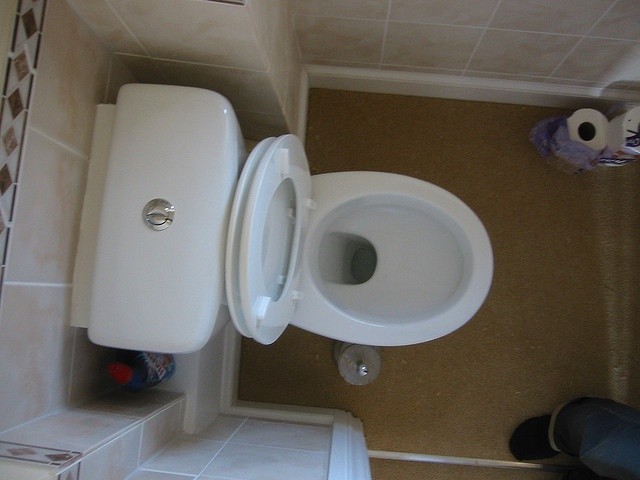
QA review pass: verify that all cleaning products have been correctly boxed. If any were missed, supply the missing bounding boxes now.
[106,354,175,392]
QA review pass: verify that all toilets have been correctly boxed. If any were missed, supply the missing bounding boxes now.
[87,83,494,356]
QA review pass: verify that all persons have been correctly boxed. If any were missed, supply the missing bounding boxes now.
[509,393,640,479]
